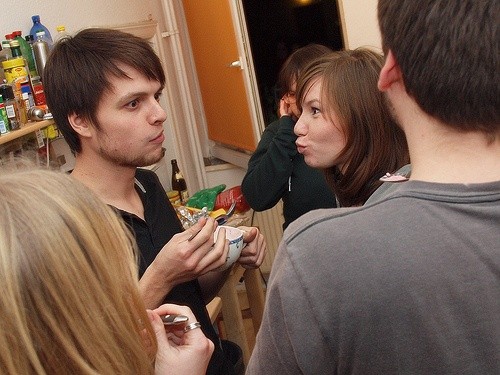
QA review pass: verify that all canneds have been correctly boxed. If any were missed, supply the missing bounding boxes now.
[0,58,36,137]
[166,191,181,220]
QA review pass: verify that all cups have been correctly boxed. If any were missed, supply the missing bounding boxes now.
[202,226,248,274]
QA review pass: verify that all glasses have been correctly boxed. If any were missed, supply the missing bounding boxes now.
[281,92,296,103]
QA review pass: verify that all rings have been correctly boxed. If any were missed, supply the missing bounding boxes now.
[183,322,201,332]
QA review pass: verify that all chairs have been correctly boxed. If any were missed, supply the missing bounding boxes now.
[200,217,267,368]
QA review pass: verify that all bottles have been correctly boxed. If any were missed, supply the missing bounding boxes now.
[33,38,51,78]
[55,26,71,47]
[35,31,55,54]
[0,31,44,133]
[170,159,189,205]
[28,15,53,57]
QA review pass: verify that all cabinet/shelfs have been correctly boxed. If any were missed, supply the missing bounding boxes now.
[0,118,55,169]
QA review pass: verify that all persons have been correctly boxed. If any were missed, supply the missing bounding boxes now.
[246,0,500,375]
[0,141,216,375]
[43,28,270,375]
[240,43,340,229]
[293,45,412,209]
